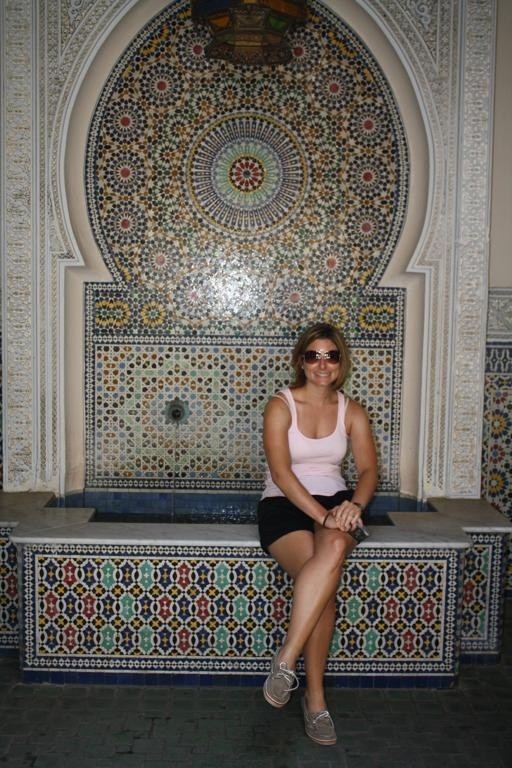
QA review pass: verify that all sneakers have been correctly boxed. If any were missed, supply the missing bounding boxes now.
[303,696,338,746]
[263,647,300,709]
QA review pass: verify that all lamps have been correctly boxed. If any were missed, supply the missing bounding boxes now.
[191,0,310,65]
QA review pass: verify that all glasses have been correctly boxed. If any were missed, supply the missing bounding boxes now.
[301,350,340,364]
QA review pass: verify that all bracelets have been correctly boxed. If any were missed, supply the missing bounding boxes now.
[322,512,331,528]
[354,502,363,510]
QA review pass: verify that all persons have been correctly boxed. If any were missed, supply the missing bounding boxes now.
[256,324,379,746]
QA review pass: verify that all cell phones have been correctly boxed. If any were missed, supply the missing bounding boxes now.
[348,525,369,544]
